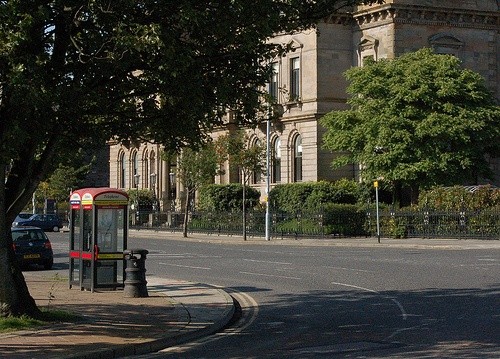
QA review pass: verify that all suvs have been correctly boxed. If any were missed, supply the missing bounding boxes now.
[16,214,63,232]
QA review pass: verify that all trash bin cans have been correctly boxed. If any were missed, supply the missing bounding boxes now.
[122,249,149,297]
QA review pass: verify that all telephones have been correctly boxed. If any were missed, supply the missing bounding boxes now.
[87,230,102,250]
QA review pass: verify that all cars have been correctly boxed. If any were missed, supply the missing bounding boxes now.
[12,213,35,227]
[10,227,54,269]
